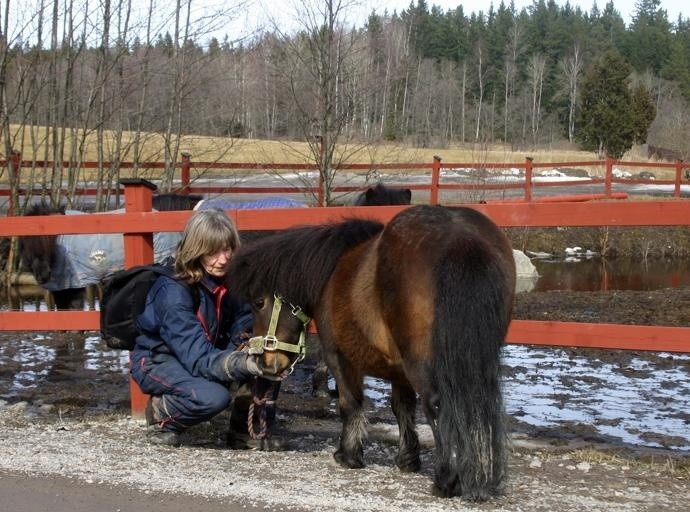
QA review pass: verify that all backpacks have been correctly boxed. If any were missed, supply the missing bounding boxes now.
[99,256,201,351]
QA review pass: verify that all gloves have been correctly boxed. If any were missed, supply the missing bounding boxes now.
[246,354,289,382]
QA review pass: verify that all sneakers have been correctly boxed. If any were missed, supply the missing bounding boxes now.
[145,394,181,448]
[227,427,290,451]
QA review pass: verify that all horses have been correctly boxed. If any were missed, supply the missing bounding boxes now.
[223,202,518,501]
[16,204,183,310]
[330,183,414,207]
[149,191,310,243]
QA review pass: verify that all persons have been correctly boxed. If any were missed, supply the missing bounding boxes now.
[130,207,289,452]
